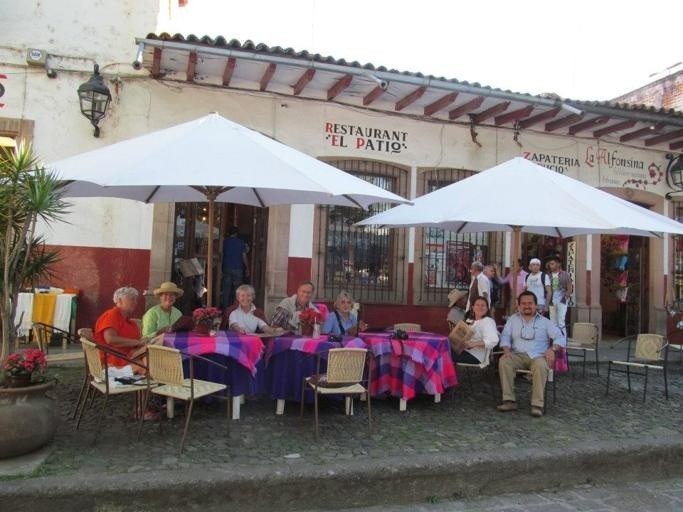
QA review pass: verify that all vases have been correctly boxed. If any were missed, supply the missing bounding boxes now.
[9,373,30,386]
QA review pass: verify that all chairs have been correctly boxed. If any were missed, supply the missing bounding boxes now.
[393,323,670,422]
[72,318,236,452]
[299,347,375,442]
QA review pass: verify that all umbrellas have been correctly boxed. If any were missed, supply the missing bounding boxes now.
[350,156,682,318]
[2,108,415,307]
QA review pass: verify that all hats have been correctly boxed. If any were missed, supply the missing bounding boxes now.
[153,281,184,298]
[528,258,541,271]
[448,288,469,308]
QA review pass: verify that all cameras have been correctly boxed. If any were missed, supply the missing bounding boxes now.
[328,335,342,342]
[394,329,408,339]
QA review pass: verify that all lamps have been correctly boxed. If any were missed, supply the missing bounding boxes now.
[77,64,112,138]
[665,152,683,200]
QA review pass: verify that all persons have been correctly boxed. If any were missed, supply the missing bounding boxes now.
[221,226,250,312]
[269,281,324,332]
[446,243,573,417]
[141,281,185,410]
[91,287,162,422]
[322,291,367,336]
[219,284,282,337]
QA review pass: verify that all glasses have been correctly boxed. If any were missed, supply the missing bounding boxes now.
[520,327,536,340]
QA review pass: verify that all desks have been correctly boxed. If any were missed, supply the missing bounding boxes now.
[14,293,79,350]
[165,330,459,426]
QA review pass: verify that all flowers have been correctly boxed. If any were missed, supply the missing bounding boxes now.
[299,302,329,322]
[3,348,47,374]
[193,308,222,323]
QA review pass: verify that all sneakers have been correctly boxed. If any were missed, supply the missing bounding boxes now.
[497,401,518,411]
[531,406,543,417]
[135,407,159,420]
